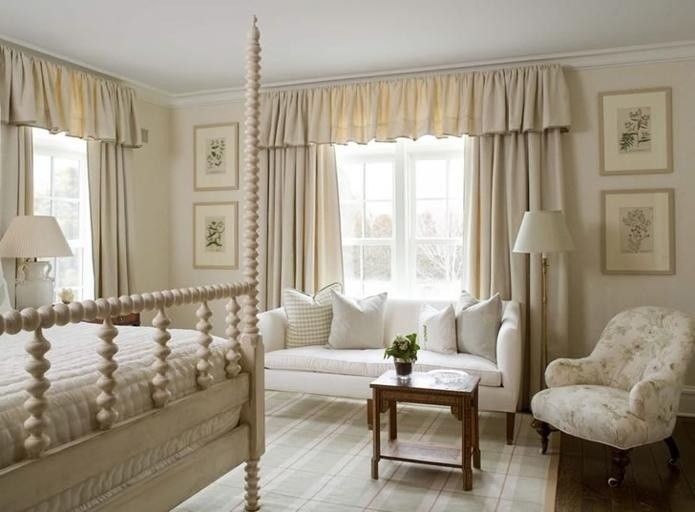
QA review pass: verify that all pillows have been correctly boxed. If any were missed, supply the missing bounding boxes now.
[279,281,390,352]
[418,287,504,365]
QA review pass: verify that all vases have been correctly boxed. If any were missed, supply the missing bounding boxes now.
[393,356,412,377]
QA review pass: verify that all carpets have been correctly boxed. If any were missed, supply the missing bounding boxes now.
[167,390,566,511]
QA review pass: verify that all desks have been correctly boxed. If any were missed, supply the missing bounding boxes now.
[368,369,483,491]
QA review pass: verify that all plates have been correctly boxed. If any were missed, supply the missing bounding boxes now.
[428,370,469,381]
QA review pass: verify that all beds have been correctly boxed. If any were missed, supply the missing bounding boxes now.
[1,14,269,512]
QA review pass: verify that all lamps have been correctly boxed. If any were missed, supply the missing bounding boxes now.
[512,208,577,390]
[2,214,73,313]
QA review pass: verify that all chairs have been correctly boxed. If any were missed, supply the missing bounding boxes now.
[529,301,695,491]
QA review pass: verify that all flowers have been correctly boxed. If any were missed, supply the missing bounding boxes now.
[383,331,420,364]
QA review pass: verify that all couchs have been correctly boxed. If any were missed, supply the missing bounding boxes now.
[253,290,527,448]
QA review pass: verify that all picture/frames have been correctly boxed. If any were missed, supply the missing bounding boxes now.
[596,85,676,178]
[191,120,241,194]
[191,201,241,270]
[598,187,678,276]
